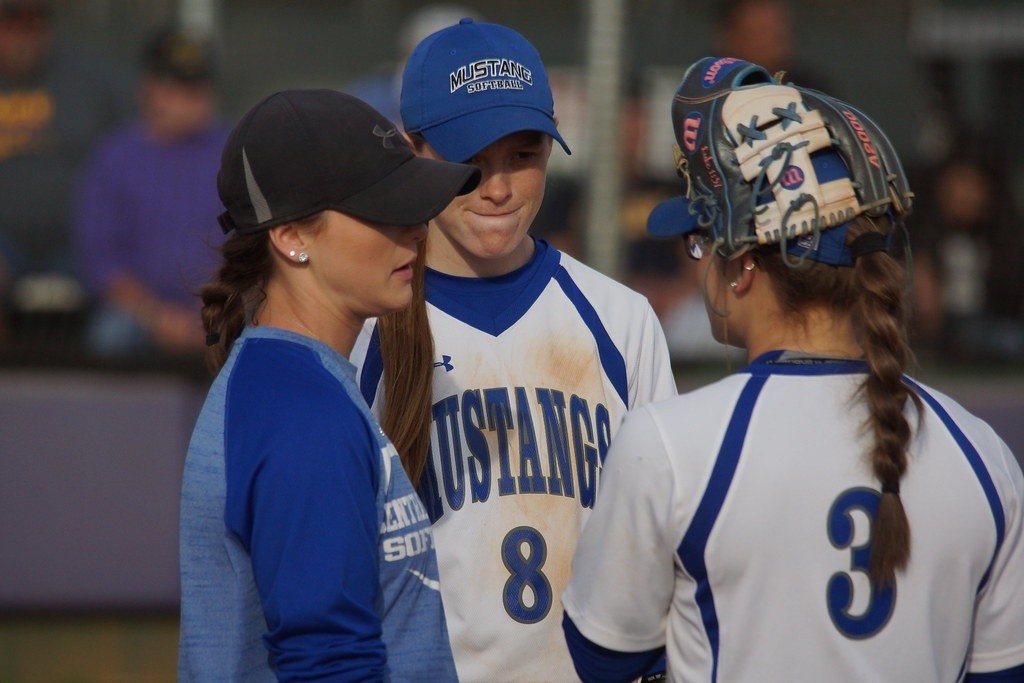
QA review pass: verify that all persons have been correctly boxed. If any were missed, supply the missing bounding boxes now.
[559,56,1024,683]
[721,1,944,350]
[79,39,245,373]
[175,88,483,683]
[350,18,677,683]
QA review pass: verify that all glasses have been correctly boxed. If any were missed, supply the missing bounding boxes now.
[683,230,712,260]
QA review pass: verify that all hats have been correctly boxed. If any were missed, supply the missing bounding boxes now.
[646,149,894,266]
[146,30,213,82]
[216,88,481,234]
[400,17,572,165]
